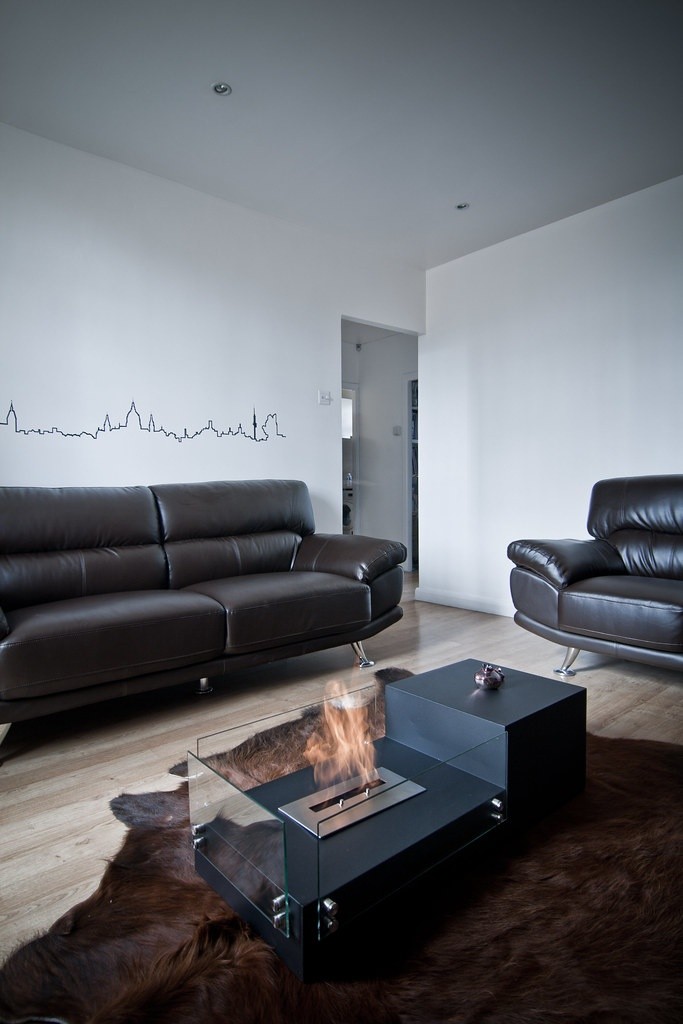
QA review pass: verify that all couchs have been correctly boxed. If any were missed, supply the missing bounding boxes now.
[504,472,683,680]
[0,477,411,744]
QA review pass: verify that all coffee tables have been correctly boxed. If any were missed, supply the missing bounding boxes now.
[184,657,598,986]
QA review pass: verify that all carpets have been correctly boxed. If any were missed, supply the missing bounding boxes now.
[0,667,683,1024]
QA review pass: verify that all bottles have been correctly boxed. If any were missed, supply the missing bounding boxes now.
[346,473,352,486]
[474,663,501,689]
